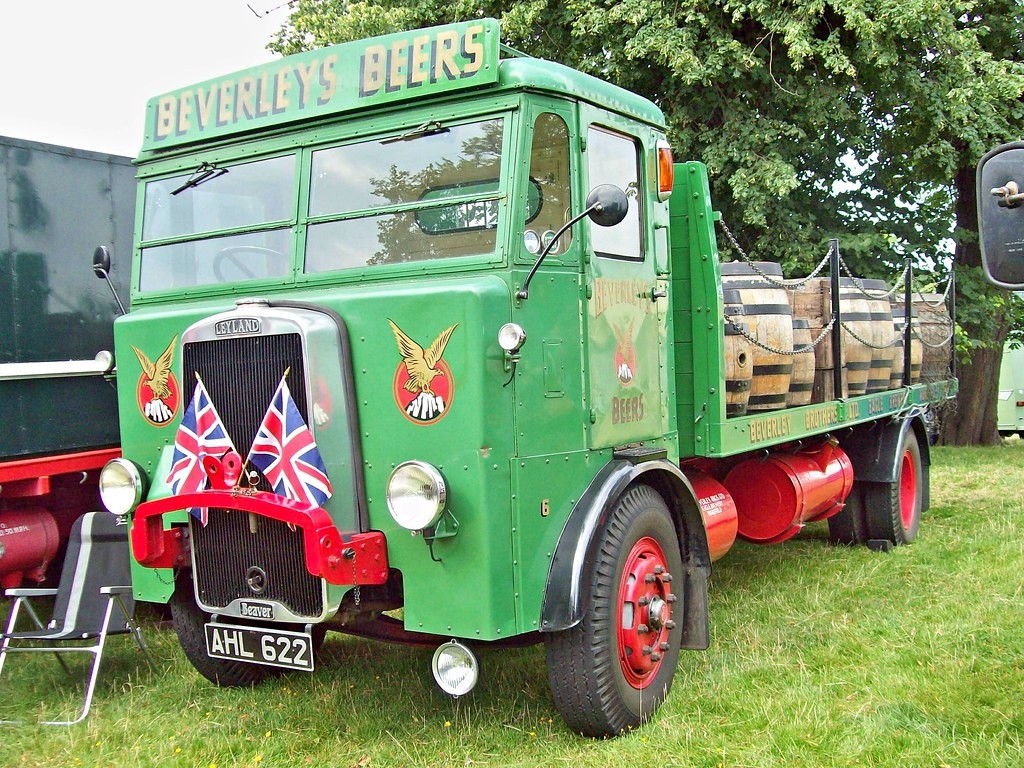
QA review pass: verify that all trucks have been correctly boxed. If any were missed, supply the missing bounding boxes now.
[93,18,959,740]
[0,137,135,597]
[960,322,1024,440]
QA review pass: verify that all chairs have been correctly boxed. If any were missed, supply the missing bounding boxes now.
[0,512,160,727]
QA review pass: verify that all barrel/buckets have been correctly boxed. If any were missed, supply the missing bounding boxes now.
[719,262,793,416]
[721,290,753,419]
[891,309,923,385]
[860,279,896,394]
[895,294,952,383]
[787,320,815,407]
[889,323,905,389]
[795,277,872,399]
